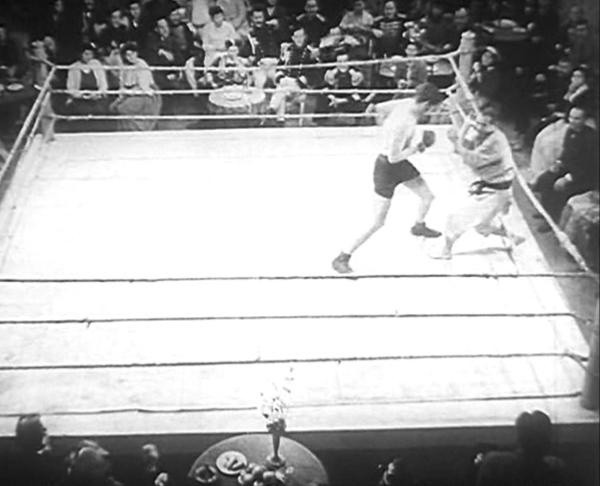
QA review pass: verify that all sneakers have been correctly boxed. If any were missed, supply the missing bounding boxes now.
[505,233,526,252]
[332,251,358,281]
[428,247,452,260]
[411,221,441,238]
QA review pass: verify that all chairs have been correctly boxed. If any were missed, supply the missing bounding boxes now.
[272,44,311,125]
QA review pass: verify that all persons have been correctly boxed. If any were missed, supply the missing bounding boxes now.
[427,107,528,261]
[331,82,444,281]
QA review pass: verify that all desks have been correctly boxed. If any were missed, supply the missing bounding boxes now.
[202,83,268,128]
[185,434,330,485]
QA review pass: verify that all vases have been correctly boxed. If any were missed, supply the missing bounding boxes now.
[265,422,290,466]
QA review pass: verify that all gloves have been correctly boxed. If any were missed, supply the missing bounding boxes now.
[418,130,435,153]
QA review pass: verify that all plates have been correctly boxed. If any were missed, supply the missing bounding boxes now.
[215,450,248,475]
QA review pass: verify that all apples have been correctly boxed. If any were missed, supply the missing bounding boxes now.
[240,463,285,486]
